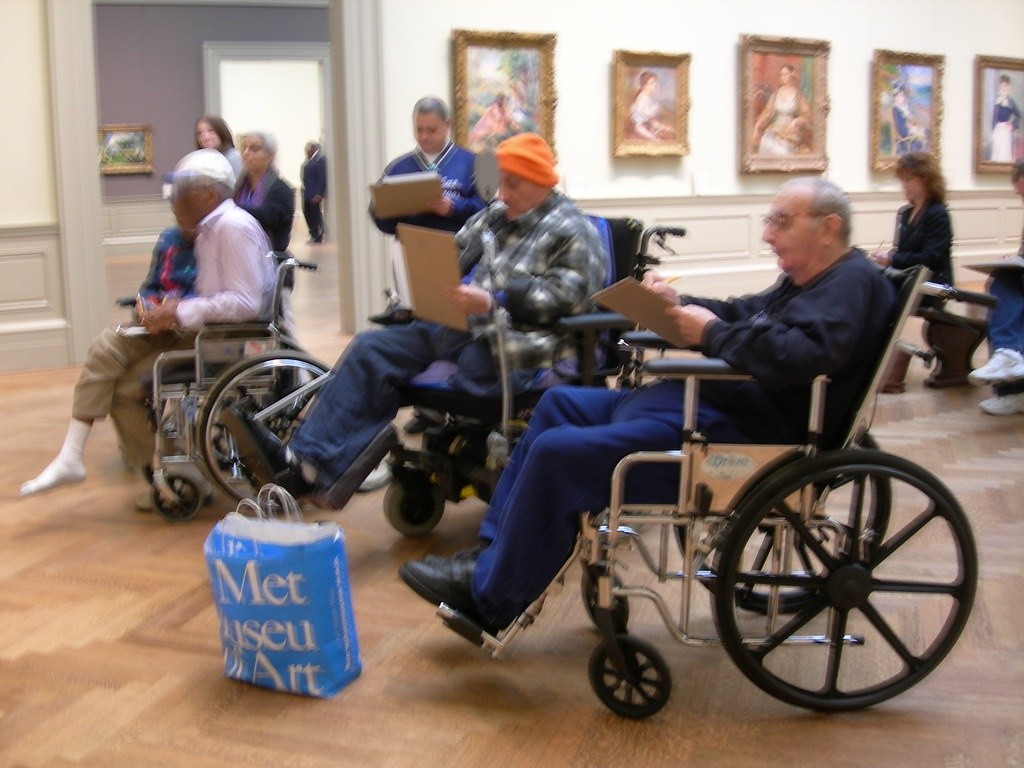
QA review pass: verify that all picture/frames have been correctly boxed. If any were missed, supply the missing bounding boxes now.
[613,47,693,157]
[739,31,831,173]
[971,53,1023,174]
[97,123,153,175]
[452,29,558,165]
[869,49,945,172]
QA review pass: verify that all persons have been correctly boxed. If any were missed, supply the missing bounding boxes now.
[368,96,487,234]
[17,146,277,511]
[299,141,326,246]
[223,134,606,512]
[230,128,295,253]
[877,151,955,310]
[195,115,243,176]
[968,160,1024,414]
[401,174,891,635]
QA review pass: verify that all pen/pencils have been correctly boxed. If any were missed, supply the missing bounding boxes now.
[161,295,166,306]
[647,276,680,291]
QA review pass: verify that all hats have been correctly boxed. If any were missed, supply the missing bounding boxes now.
[496,132,557,188]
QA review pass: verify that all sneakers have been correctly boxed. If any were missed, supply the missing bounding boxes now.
[968,347,1024,386]
[980,392,1024,415]
[424,545,483,569]
[400,559,499,637]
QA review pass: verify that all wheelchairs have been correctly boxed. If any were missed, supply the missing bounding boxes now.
[434,259,1003,722]
[212,214,692,549]
[114,249,338,524]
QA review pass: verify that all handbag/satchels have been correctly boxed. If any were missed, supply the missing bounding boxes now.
[205,483,362,700]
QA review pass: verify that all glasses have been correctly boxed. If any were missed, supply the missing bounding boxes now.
[764,211,848,232]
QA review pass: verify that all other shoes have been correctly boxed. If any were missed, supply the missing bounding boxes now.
[357,456,393,490]
[403,414,436,434]
[224,406,310,505]
